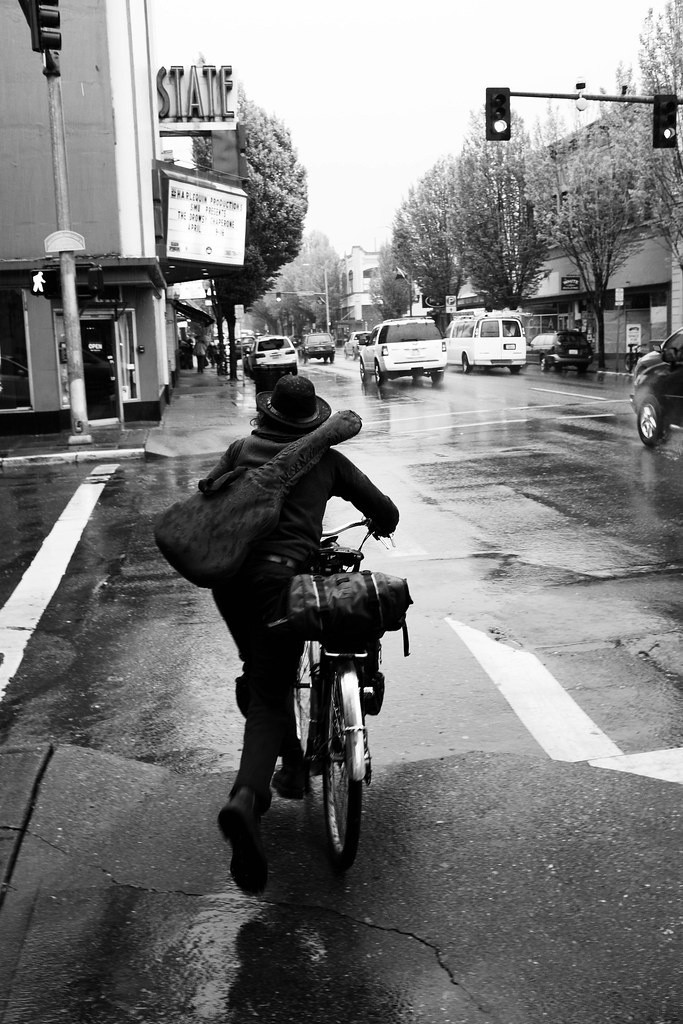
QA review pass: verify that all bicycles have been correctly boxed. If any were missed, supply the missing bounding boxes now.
[294,514,383,871]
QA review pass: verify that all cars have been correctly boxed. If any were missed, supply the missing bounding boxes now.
[344,331,373,361]
[1,354,31,405]
[290,334,303,356]
[302,333,335,363]
[630,347,683,447]
[246,336,298,379]
[58,347,114,402]
[235,333,262,372]
[632,326,683,380]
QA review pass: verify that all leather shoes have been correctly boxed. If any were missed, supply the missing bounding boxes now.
[271,739,315,803]
[216,786,275,897]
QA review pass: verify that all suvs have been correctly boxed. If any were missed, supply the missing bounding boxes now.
[526,330,593,375]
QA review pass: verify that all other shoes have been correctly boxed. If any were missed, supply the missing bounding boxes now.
[198,370,203,375]
[218,372,229,377]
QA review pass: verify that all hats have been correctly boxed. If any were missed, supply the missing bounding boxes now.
[255,375,333,439]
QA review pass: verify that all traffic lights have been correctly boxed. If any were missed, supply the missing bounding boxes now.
[29,269,59,296]
[485,87,512,142]
[652,93,679,148]
[276,291,282,303]
[33,0,64,53]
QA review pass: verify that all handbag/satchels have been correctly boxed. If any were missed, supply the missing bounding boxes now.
[288,570,414,652]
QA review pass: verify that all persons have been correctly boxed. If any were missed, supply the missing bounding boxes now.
[178,338,218,374]
[204,377,400,896]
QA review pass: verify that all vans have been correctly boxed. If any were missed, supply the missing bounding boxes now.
[359,317,447,385]
[444,316,527,374]
